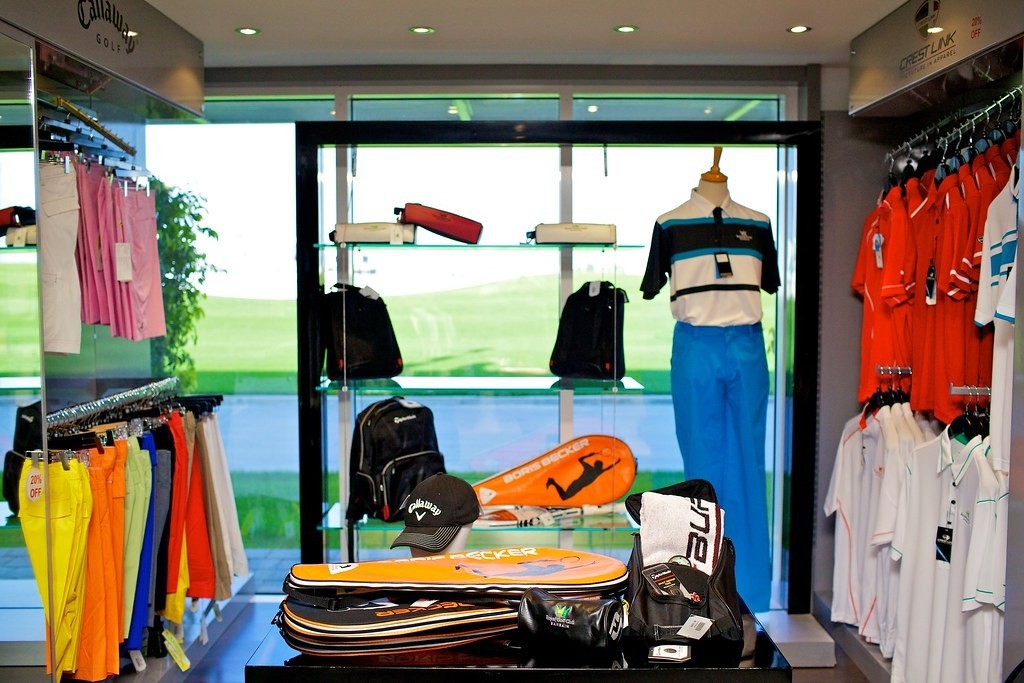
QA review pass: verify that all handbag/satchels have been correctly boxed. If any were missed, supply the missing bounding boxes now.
[626,479,745,667]
[329,221,417,245]
[394,202,483,244]
[526,223,616,244]
[517,586,624,652]
[549,281,630,380]
[322,283,403,380]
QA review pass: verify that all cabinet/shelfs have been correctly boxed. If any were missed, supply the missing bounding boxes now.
[292,121,836,675]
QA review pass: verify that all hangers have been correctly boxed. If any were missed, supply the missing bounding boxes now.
[947,386,993,447]
[866,362,916,417]
[37,100,155,197]
[21,379,224,471]
[880,91,1023,197]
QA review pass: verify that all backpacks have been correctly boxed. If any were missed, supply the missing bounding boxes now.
[344,395,447,522]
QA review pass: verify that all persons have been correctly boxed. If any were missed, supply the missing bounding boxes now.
[638,147,782,615]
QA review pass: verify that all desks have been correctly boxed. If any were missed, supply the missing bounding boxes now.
[246,597,792,681]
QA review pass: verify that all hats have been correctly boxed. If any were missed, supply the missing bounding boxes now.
[390,473,479,553]
